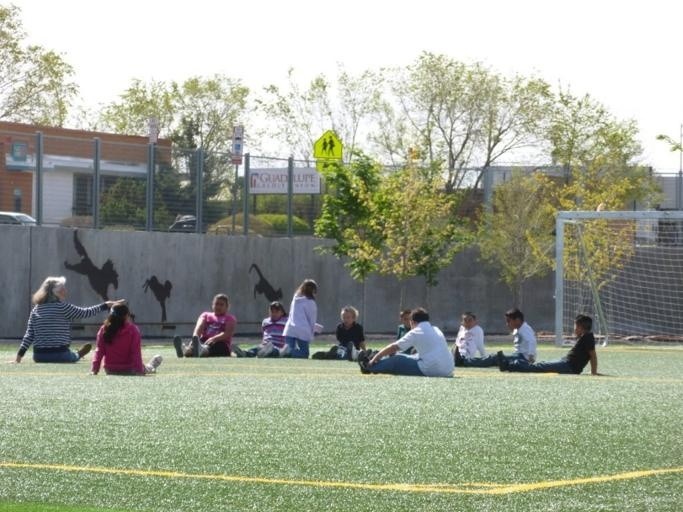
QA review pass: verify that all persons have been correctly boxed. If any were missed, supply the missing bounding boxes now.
[453,308,536,367]
[266,278,318,358]
[375,310,415,355]
[452,313,483,358]
[231,301,289,358]
[336,305,366,360]
[357,308,454,377]
[173,293,237,358]
[16,276,125,363]
[89,304,162,374]
[498,314,600,374]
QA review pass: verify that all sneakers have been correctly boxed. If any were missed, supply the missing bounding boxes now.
[499,351,509,371]
[191,335,199,357]
[174,335,185,358]
[257,341,271,358]
[78,343,91,357]
[347,341,357,361]
[231,343,245,357]
[454,346,463,367]
[358,351,369,373]
[145,354,163,372]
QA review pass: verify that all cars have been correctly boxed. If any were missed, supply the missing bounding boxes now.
[0,210,39,227]
[167,213,199,234]
